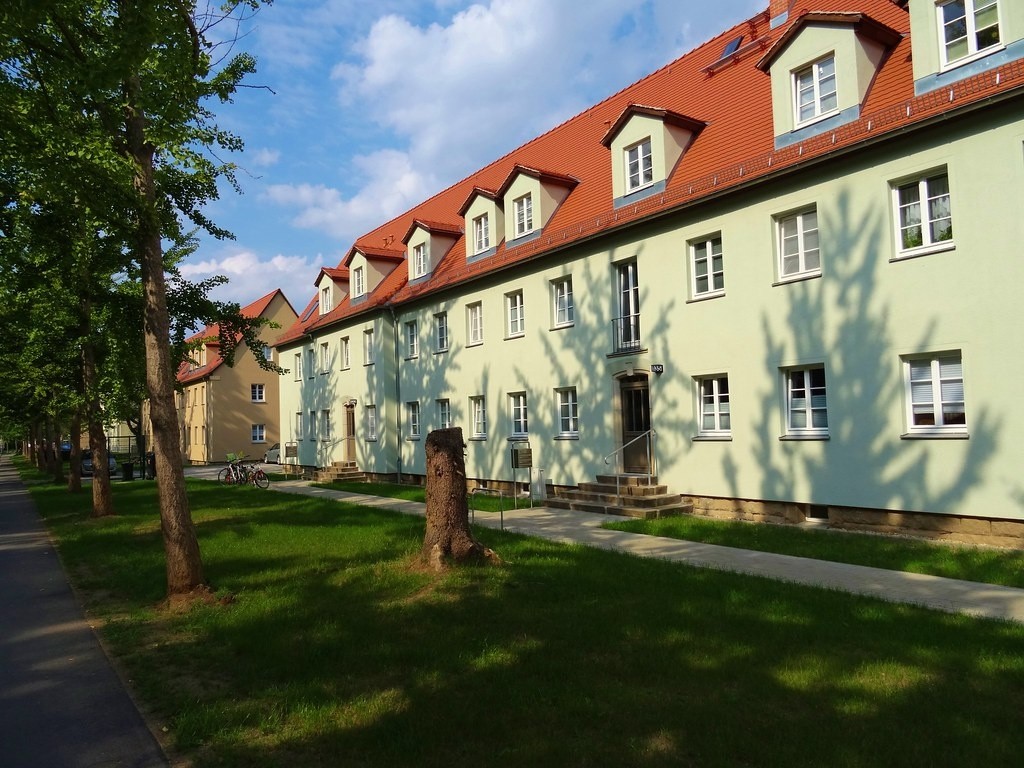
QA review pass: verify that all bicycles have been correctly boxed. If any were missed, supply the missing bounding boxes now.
[218,455,269,489]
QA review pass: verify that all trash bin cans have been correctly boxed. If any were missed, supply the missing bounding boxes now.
[121,461,135,481]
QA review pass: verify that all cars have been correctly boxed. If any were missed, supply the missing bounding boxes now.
[264,443,280,464]
[60,442,71,457]
[81,448,117,476]
